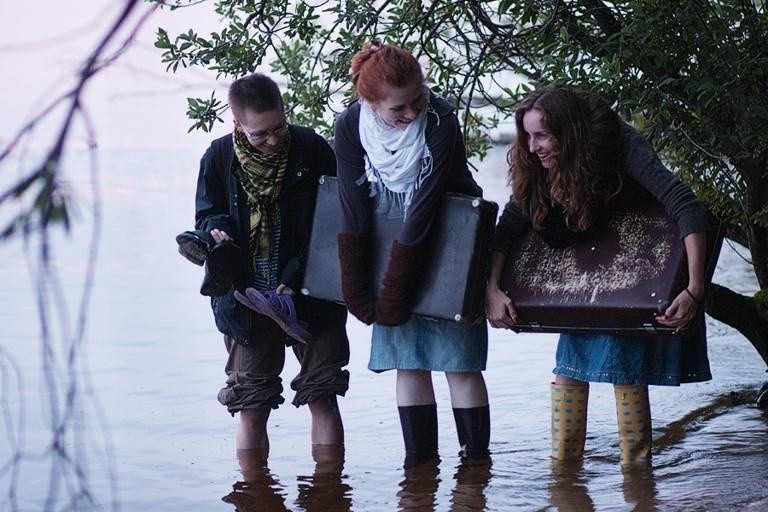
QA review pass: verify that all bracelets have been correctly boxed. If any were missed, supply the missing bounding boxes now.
[685,287,697,305]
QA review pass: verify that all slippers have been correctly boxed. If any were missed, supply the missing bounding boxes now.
[245,287,311,344]
[200,243,245,296]
[233,290,308,330]
[175,230,214,266]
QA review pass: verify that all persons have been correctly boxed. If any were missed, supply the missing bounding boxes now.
[176,73,350,451]
[334,38,491,470]
[234,450,344,481]
[488,86,710,467]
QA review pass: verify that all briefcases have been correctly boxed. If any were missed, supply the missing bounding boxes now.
[300,176,500,327]
[496,208,726,336]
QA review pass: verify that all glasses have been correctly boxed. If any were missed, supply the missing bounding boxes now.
[240,118,290,142]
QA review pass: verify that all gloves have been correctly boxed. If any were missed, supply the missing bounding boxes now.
[337,231,376,325]
[375,240,427,326]
